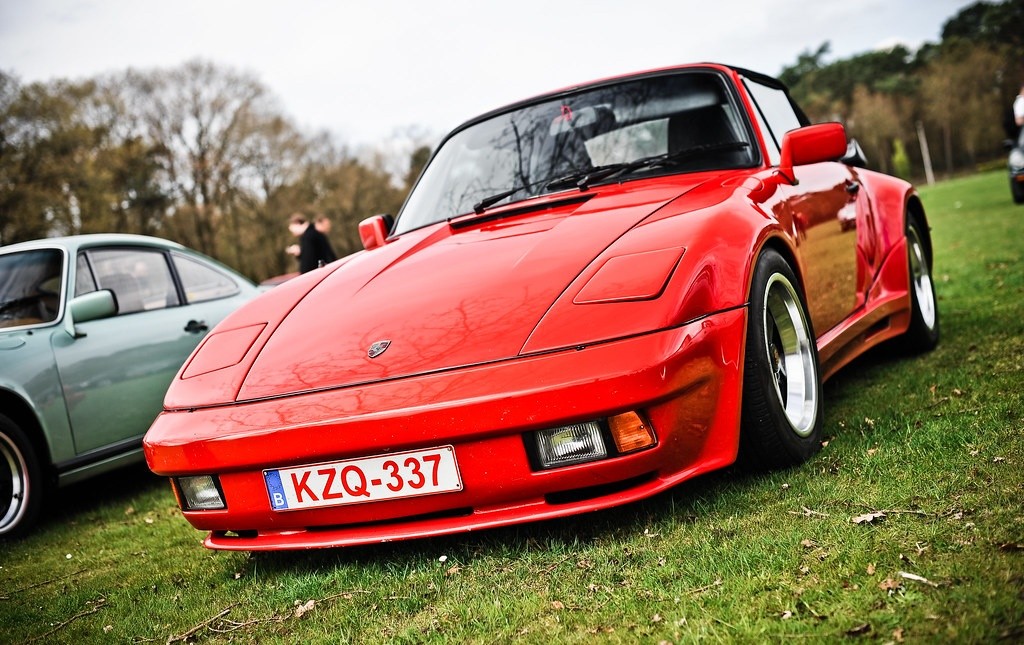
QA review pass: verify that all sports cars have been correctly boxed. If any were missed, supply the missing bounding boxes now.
[142,61,941,550]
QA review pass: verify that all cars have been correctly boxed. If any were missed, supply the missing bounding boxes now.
[75,364,125,389]
[1002,124,1024,205]
[837,200,858,230]
[0,232,256,534]
[34,382,73,401]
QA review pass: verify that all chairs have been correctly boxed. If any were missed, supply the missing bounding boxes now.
[99,271,145,313]
[522,128,592,198]
[666,103,752,166]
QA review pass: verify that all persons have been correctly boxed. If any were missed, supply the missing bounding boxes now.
[285,213,336,275]
